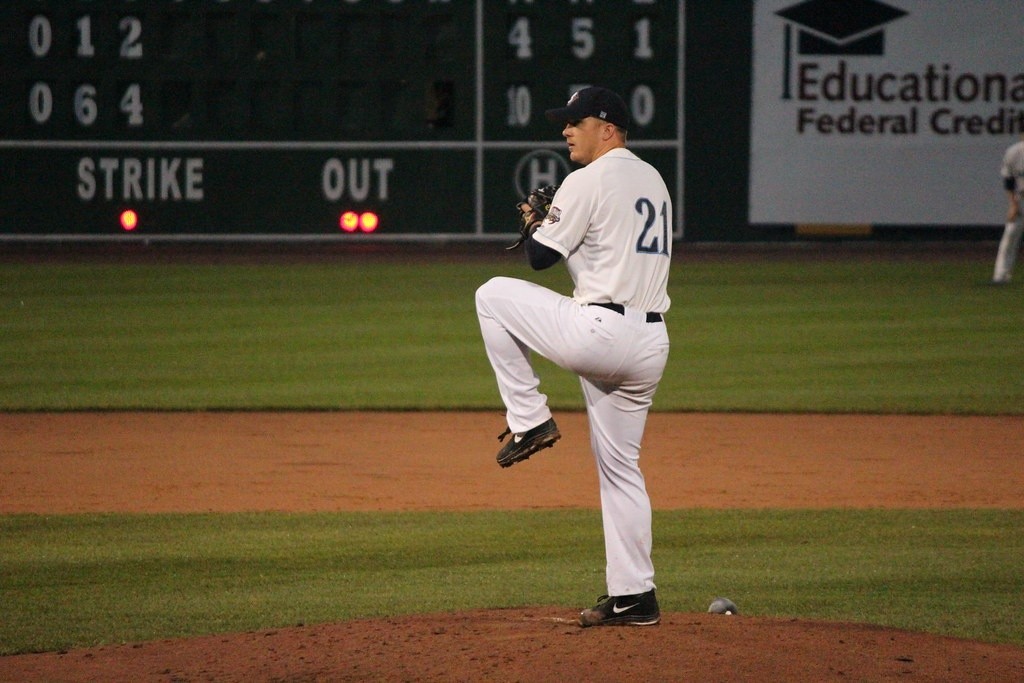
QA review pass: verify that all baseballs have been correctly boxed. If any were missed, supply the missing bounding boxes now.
[708,597,738,616]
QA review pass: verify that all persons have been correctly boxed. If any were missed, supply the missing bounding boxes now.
[993,125,1024,285]
[475,87,673,626]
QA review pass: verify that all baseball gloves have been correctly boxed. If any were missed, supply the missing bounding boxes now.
[505,185,559,251]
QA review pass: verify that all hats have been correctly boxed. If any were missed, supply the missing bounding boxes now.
[544,87,629,131]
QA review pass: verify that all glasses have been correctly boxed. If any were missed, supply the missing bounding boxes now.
[558,113,595,128]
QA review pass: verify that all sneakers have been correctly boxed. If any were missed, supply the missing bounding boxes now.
[496,417,561,468]
[578,589,661,628]
